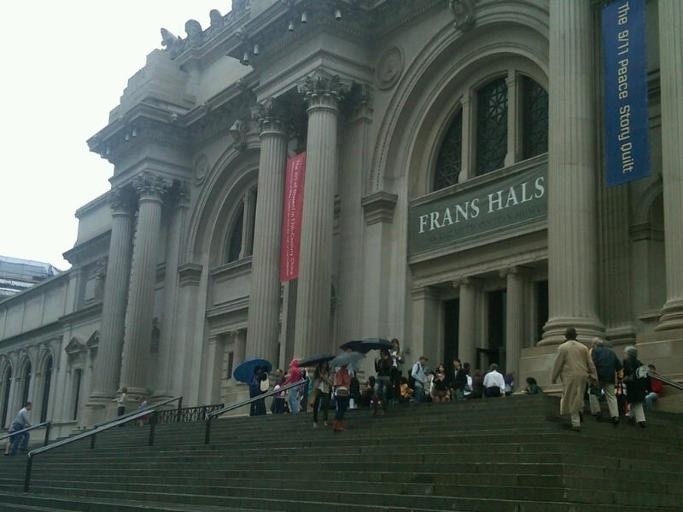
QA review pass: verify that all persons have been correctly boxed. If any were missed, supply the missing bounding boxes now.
[116,386,127,429]
[586,335,622,426]
[549,326,597,432]
[135,394,149,428]
[243,337,513,435]
[622,344,649,428]
[3,417,16,456]
[9,402,33,458]
[521,376,543,394]
[623,364,665,418]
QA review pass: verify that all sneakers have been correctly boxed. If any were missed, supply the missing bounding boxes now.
[313,421,328,428]
[629,417,646,428]
[596,412,620,424]
[570,411,583,431]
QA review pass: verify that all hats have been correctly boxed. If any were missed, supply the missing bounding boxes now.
[561,328,578,335]
[624,346,637,358]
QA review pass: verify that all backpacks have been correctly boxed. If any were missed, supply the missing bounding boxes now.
[636,365,647,379]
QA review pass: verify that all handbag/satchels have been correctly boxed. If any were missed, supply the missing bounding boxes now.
[616,393,625,406]
[408,368,415,390]
[260,379,269,391]
[623,373,635,383]
[310,390,317,407]
[331,386,350,400]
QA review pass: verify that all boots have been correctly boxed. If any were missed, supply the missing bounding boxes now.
[332,420,347,431]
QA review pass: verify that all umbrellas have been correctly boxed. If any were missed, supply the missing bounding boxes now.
[231,357,272,384]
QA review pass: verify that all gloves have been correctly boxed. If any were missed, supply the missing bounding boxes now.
[617,368,624,380]
